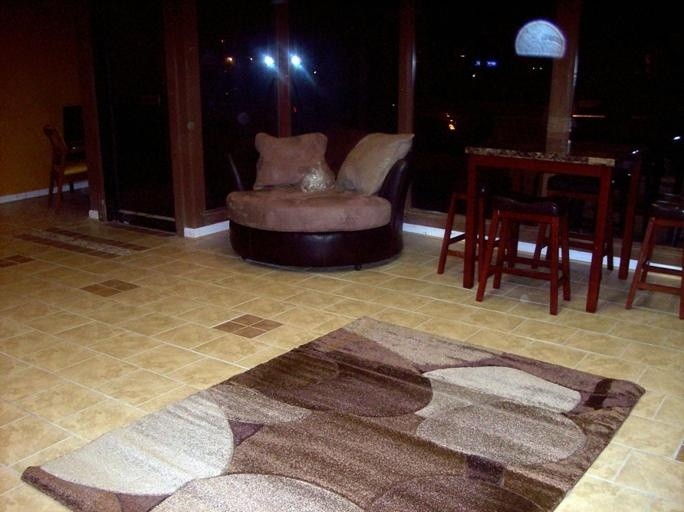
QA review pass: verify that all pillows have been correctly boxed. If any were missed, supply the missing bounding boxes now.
[253,132,414,198]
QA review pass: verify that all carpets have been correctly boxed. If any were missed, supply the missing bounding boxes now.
[22,315,646,511]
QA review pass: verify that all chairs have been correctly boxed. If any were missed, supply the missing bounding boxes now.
[224,121,424,269]
[43,124,88,216]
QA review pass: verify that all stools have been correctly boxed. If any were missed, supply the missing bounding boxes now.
[438,175,684,320]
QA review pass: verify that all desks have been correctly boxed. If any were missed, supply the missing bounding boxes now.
[459,129,651,316]
